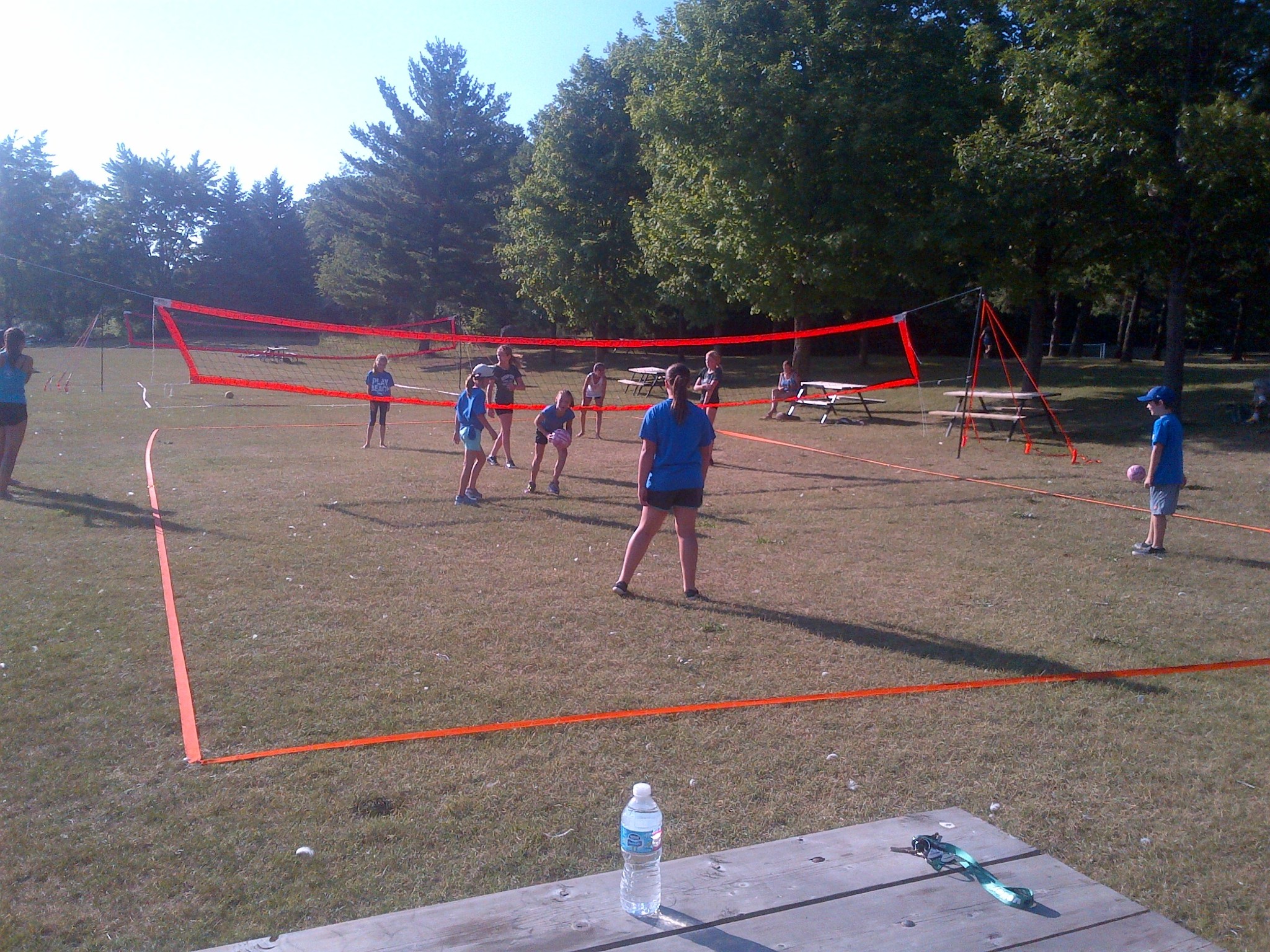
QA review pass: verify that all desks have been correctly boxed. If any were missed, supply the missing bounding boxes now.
[205,806,1224,952]
[787,381,873,424]
[624,367,665,398]
[267,346,289,363]
[943,391,1062,442]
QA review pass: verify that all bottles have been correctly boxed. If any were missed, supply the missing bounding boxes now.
[620,783,663,919]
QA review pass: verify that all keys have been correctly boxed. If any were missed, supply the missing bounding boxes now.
[890,833,942,854]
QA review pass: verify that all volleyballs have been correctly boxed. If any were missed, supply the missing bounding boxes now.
[1180,473,1187,488]
[776,412,784,420]
[551,429,571,448]
[225,392,233,398]
[1127,465,1146,481]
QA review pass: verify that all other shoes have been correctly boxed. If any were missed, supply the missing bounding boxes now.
[486,455,500,466]
[505,458,516,468]
[465,488,483,499]
[454,495,478,506]
[683,589,699,601]
[1256,400,1268,408]
[612,580,627,595]
[1245,416,1261,423]
[1132,546,1166,555]
[547,481,560,494]
[1134,539,1152,550]
[524,481,536,493]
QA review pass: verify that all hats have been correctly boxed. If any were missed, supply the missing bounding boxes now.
[472,363,499,380]
[1136,386,1175,402]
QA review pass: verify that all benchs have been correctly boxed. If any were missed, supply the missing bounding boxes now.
[828,395,887,404]
[285,352,299,362]
[928,410,1027,422]
[976,405,1073,414]
[796,400,831,406]
[617,379,651,387]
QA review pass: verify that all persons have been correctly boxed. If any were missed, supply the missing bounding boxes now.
[360,353,394,449]
[1245,380,1270,424]
[1131,385,1187,559]
[0,327,33,502]
[693,350,723,466]
[763,360,801,419]
[575,362,607,440]
[452,363,499,508]
[980,323,993,360]
[485,344,526,468]
[524,389,575,497]
[611,364,716,601]
